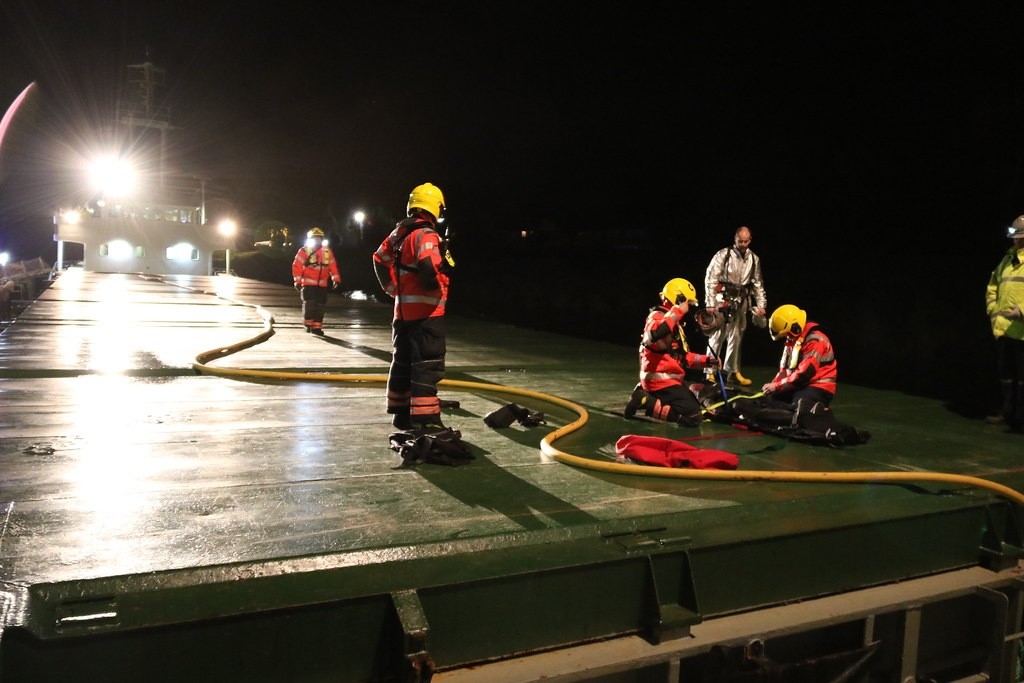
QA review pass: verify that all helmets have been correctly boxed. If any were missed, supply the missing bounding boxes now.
[769,304,807,340]
[659,278,699,309]
[407,182,447,220]
[308,227,325,237]
[1007,215,1024,237]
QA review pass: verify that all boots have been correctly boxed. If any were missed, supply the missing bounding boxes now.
[729,372,753,386]
[306,321,324,335]
[988,379,1023,431]
[707,373,715,383]
[392,409,461,441]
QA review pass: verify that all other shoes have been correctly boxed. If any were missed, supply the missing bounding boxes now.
[624,386,653,419]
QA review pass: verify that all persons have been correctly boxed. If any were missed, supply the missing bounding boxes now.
[372,183,455,434]
[624,278,722,427]
[985,215,1024,434]
[705,226,767,385]
[291,227,341,335]
[762,304,838,414]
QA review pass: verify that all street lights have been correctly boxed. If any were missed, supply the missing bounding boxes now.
[221,221,234,273]
[354,212,366,239]
